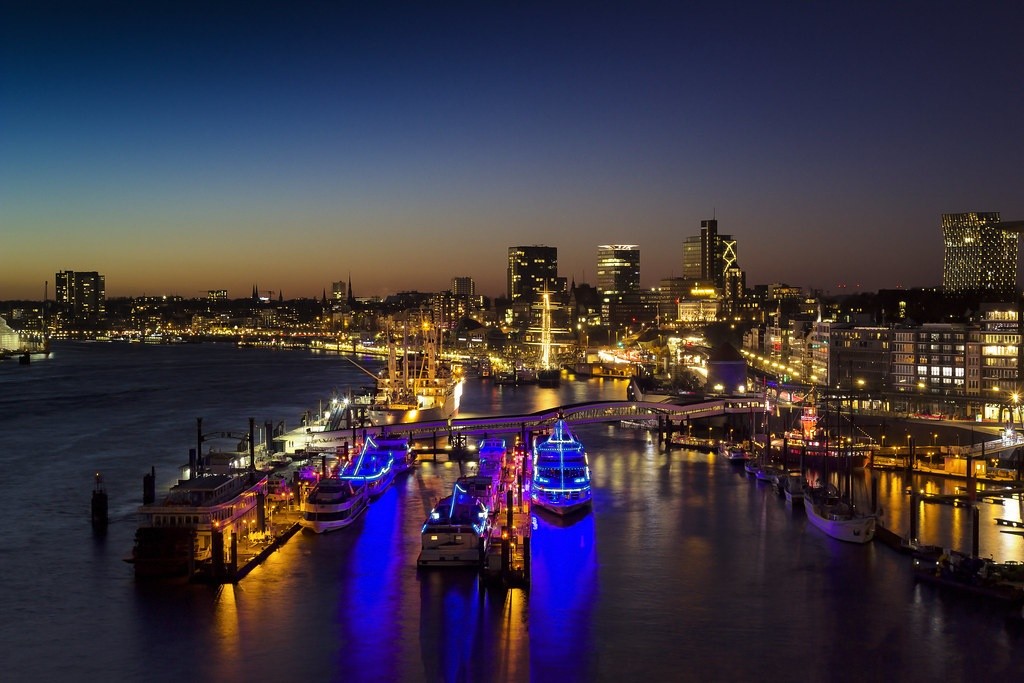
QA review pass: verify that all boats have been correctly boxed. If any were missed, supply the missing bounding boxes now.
[417,434,506,576]
[531,411,591,515]
[126,326,466,593]
[143,329,186,344]
[468,350,535,387]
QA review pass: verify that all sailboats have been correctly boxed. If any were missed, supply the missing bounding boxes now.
[619,370,877,546]
[524,282,573,388]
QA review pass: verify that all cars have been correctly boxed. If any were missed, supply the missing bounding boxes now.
[932,412,943,418]
